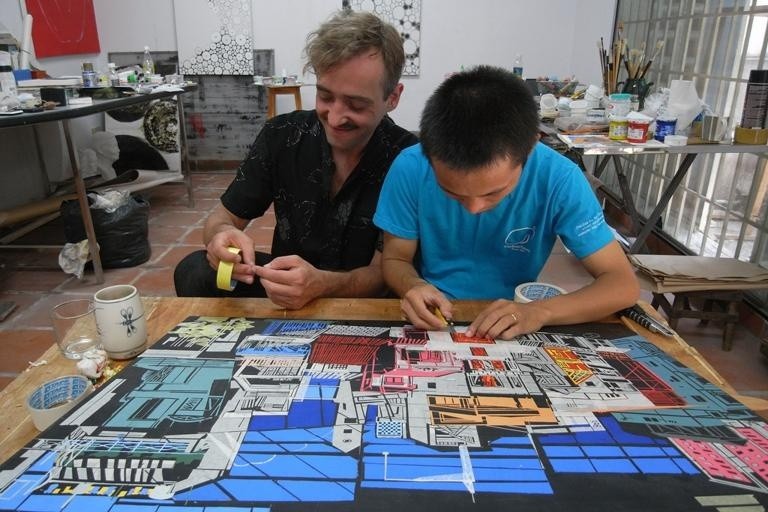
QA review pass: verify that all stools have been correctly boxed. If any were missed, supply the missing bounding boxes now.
[266,83,302,116]
[650,287,742,351]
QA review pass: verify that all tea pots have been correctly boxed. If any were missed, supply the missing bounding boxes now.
[616,78,654,111]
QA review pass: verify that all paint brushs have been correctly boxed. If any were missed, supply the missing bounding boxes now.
[599,22,664,100]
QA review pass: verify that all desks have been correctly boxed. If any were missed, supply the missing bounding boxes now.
[537,111,766,253]
[2,295,767,512]
[0,81,197,283]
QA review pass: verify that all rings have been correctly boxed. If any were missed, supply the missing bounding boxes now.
[511,314,515,322]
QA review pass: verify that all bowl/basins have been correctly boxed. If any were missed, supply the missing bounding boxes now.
[664,136,688,146]
[734,126,767,145]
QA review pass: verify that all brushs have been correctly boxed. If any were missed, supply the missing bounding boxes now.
[562,126,610,135]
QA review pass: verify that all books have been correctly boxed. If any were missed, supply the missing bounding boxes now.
[253,73,298,86]
[68,97,93,108]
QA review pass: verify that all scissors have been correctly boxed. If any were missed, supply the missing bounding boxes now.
[435,307,457,336]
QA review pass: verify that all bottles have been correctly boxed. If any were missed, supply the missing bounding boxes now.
[81,71,96,89]
[653,117,677,142]
[513,60,523,78]
[143,48,155,76]
[82,62,92,72]
[628,119,649,143]
[41,86,66,107]
[106,63,121,86]
[632,95,639,112]
[607,118,627,140]
[740,70,768,130]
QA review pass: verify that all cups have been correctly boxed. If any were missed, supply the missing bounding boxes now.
[124,67,184,87]
[702,111,719,142]
[540,85,606,122]
[254,76,296,87]
[93,284,146,361]
[32,70,47,79]
[50,298,100,358]
[609,94,630,116]
[14,70,30,85]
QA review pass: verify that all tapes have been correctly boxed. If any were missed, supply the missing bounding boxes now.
[216,247,243,292]
[513,282,569,304]
[27,375,95,432]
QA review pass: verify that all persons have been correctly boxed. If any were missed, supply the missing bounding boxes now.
[372,64,638,339]
[174,7,420,311]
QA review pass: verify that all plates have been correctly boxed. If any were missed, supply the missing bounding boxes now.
[13,108,44,112]
[0,111,23,114]
